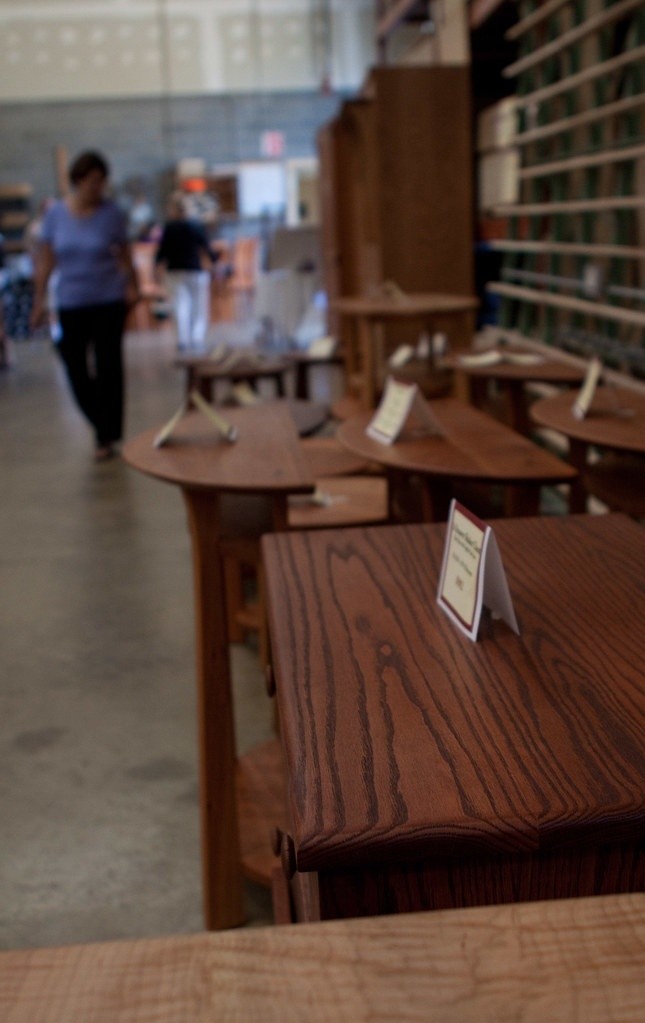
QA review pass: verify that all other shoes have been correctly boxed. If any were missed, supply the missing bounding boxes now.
[93,445,112,460]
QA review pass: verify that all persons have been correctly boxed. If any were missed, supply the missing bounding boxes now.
[154,197,217,357]
[30,152,144,460]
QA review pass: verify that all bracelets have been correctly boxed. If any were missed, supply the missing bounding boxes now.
[132,297,142,306]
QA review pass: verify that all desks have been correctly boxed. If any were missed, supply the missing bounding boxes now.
[1,851,644,1023]
[120,296,645,931]
